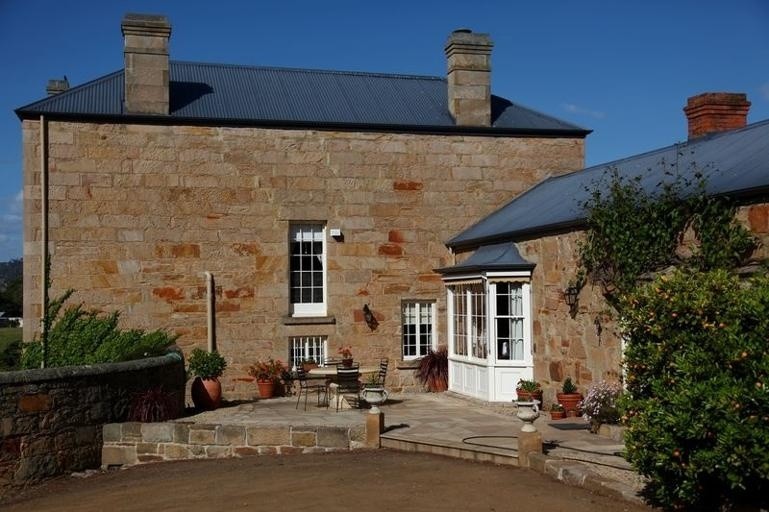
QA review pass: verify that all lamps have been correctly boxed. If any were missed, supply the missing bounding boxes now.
[564,285,578,319]
[363,304,373,322]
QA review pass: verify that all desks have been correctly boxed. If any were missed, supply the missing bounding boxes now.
[308,366,384,409]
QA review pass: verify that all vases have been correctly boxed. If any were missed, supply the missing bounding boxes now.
[342,359,353,367]
[257,383,276,398]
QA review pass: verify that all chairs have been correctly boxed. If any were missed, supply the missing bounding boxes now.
[361,358,389,400]
[324,362,360,412]
[295,363,330,412]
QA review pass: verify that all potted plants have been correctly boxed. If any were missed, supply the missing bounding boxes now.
[550,403,565,419]
[412,344,448,392]
[556,378,584,417]
[567,408,577,417]
[186,347,227,410]
[280,370,298,397]
[516,378,543,401]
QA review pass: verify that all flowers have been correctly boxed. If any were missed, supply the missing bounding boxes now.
[337,345,352,359]
[247,357,285,382]
